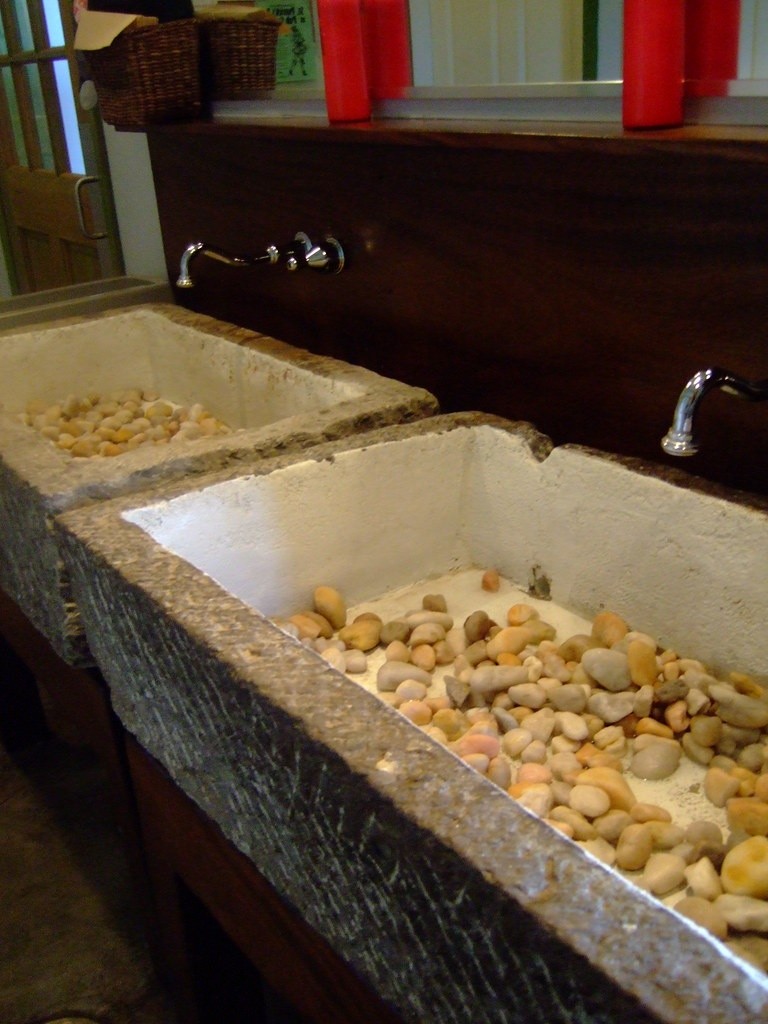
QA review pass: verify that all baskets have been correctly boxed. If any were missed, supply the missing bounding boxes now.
[83,17,207,129]
[202,13,283,99]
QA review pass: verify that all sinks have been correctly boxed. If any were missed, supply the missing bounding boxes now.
[48,406,768,1023]
[0,302,442,670]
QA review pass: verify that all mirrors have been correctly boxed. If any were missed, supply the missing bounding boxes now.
[193,0,325,101]
[363,0,624,100]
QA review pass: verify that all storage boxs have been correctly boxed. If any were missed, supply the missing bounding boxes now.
[0,299,438,675]
[81,17,208,126]
[51,409,766,1022]
[201,19,281,102]
[0,274,172,330]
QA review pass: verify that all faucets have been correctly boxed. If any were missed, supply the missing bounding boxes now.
[176,228,350,289]
[661,368,768,458]
